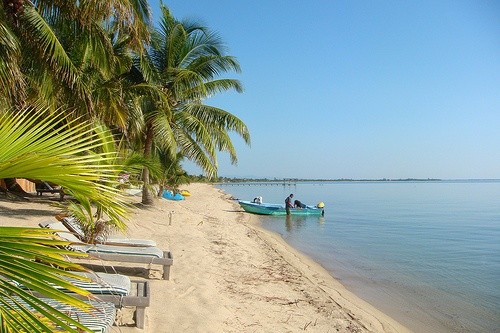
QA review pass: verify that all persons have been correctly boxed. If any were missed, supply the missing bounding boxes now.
[294,200,310,208]
[285,194,294,215]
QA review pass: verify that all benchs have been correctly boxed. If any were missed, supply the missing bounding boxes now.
[5,271,151,330]
[39,220,173,281]
[54,214,156,247]
[1,295,117,333]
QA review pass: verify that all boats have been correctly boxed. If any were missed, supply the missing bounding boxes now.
[238,200,323,216]
